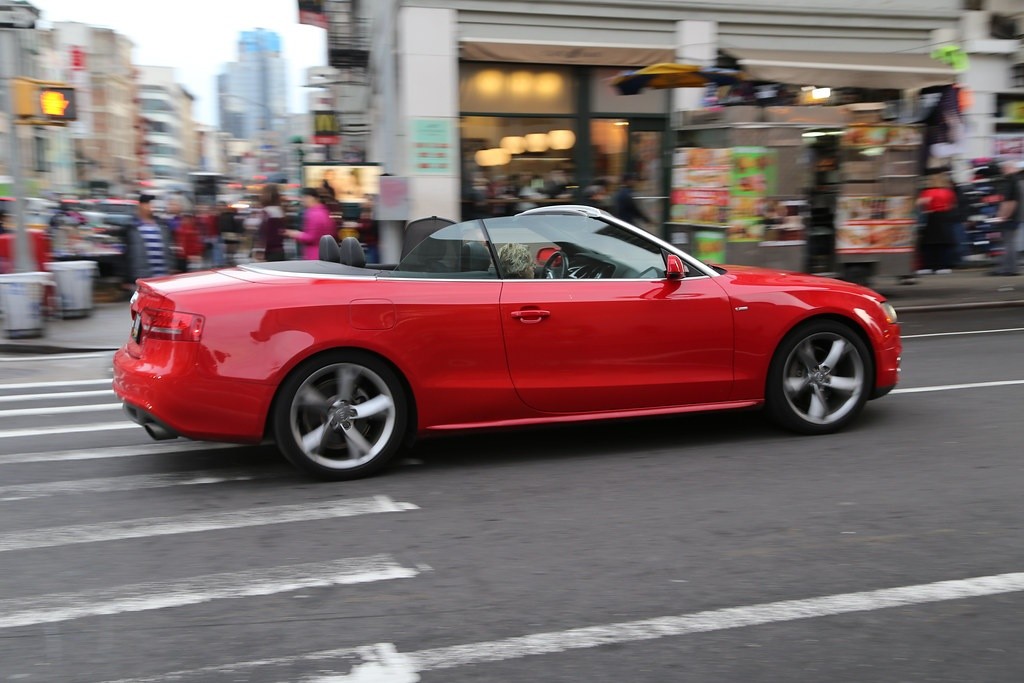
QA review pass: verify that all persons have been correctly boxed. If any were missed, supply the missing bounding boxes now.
[324,168,363,198]
[119,185,237,279]
[995,161,1024,276]
[914,175,958,274]
[256,183,336,259]
[498,243,535,279]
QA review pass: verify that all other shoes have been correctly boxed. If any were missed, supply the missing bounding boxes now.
[916,268,951,275]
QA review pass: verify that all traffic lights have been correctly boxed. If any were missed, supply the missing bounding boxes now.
[40,87,76,120]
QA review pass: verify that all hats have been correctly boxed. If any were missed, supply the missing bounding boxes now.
[139,195,155,203]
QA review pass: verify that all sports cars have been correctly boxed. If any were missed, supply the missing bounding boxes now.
[113,205,903,480]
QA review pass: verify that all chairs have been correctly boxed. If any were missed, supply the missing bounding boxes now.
[339,237,367,269]
[318,234,339,262]
[460,241,492,272]
